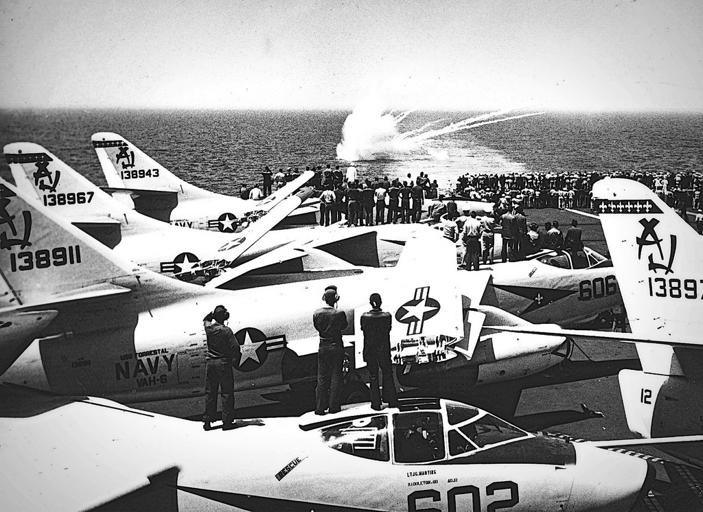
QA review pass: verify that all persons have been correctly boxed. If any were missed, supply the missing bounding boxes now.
[623,168,702,235]
[456,170,623,210]
[426,195,564,269]
[312,288,347,416]
[345,163,357,184]
[564,218,585,251]
[249,184,265,200]
[203,305,240,431]
[319,171,438,227]
[360,292,402,411]
[240,184,250,200]
[273,167,300,187]
[305,164,344,190]
[262,166,273,196]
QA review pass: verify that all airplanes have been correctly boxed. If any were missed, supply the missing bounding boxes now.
[480,178,703,469]
[0,141,542,279]
[0,176,574,406]
[90,131,515,233]
[300,248,627,333]
[1,309,656,512]
